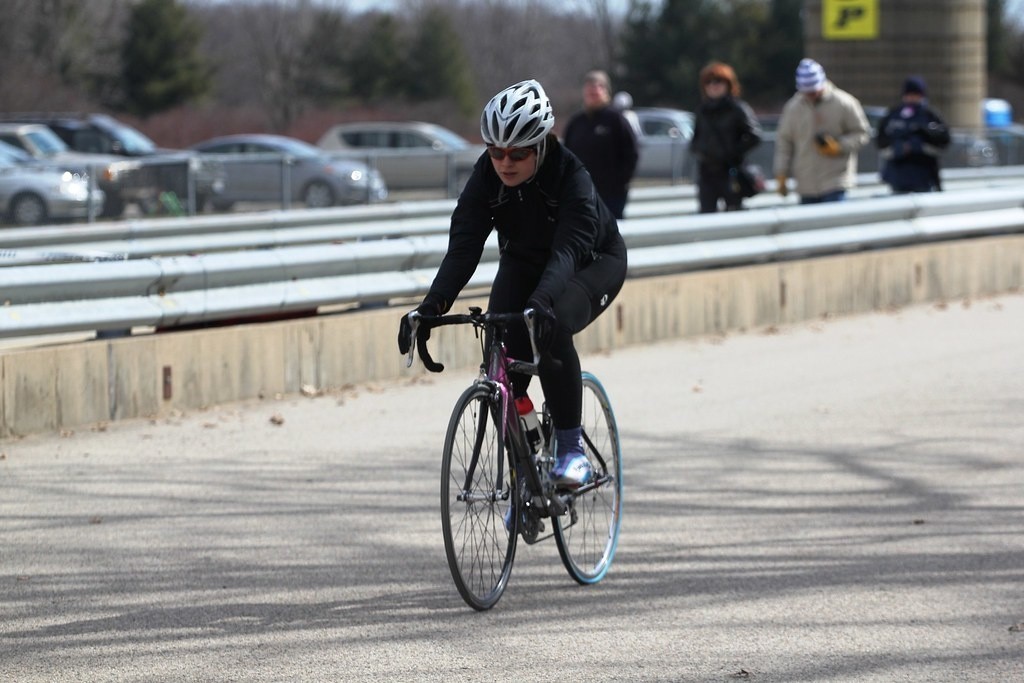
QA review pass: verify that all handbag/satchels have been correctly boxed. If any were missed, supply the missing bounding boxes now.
[735,165,764,198]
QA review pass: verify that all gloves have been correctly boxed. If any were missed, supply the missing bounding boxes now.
[813,136,839,157]
[398,295,443,354]
[522,296,556,354]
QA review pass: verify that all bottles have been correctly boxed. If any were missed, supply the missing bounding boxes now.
[515,396,546,452]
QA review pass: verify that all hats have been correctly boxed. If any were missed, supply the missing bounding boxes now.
[796,58,825,91]
[904,77,926,96]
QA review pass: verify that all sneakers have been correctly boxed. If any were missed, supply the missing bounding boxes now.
[549,448,591,484]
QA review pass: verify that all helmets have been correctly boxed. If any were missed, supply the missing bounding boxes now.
[480,79,555,149]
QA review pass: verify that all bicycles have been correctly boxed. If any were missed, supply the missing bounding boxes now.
[401,307,627,611]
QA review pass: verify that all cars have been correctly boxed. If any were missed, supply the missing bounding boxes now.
[754,104,1024,176]
[319,121,489,197]
[0,122,135,217]
[120,131,390,209]
[632,109,707,181]
[0,140,109,227]
[4,107,174,157]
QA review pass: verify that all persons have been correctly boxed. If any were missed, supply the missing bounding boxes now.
[775,58,870,204]
[398,79,627,526]
[564,73,638,218]
[689,63,762,213]
[875,76,952,194]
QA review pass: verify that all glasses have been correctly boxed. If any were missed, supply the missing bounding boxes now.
[487,145,533,161]
[705,79,722,84]
